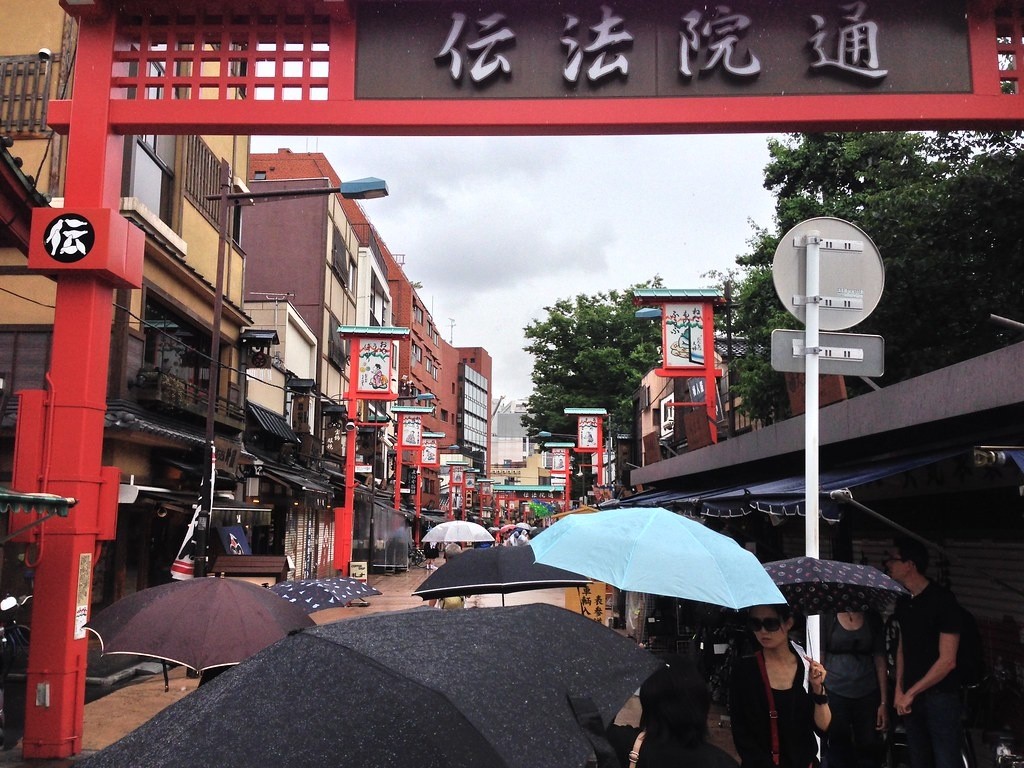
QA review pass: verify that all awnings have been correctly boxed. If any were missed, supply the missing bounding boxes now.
[324,467,373,496]
[367,483,394,498]
[243,464,334,509]
[0,488,80,544]
[422,509,446,517]
[586,432,1024,596]
[149,493,272,512]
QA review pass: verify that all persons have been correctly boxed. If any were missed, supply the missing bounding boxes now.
[465,511,550,547]
[819,608,888,768]
[635,668,740,768]
[885,537,979,768]
[728,603,840,768]
[423,542,471,609]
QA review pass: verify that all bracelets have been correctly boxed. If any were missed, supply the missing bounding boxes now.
[881,701,886,705]
[811,690,827,705]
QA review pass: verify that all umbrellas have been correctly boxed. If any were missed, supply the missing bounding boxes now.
[499,523,517,533]
[81,576,318,673]
[515,522,532,530]
[64,601,666,768]
[412,544,595,601]
[421,519,495,541]
[529,504,788,610]
[760,556,913,614]
[264,576,383,614]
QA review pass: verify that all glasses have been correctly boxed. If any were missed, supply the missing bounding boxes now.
[747,617,786,631]
[884,556,901,563]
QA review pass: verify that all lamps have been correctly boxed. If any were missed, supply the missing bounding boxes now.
[345,419,355,431]
[38,49,51,61]
